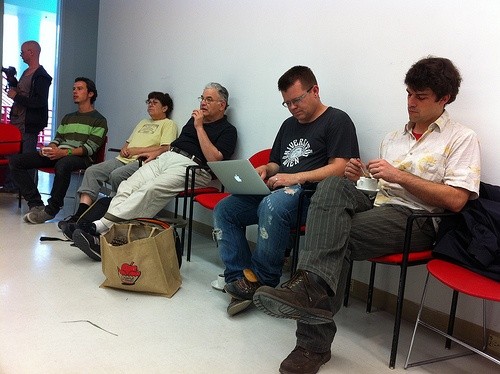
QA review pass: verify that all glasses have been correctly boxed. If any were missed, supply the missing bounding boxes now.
[282,86,313,107]
[146,100,164,107]
[198,97,223,104]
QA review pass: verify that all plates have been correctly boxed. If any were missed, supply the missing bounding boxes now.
[355,186,380,192]
[212,280,224,290]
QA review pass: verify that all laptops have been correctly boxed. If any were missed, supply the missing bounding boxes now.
[206,158,282,195]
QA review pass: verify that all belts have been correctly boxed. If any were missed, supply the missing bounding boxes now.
[170,147,213,175]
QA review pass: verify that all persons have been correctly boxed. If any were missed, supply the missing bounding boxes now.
[8,40,53,210]
[213,66,360,315]
[8,76,108,224]
[58,91,178,229]
[72,83,238,263]
[253,58,479,374]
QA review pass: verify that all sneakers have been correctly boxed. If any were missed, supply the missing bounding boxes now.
[279,344,331,374]
[63,222,101,241]
[72,229,101,261]
[253,269,333,324]
[224,269,265,300]
[27,207,55,223]
[227,297,252,315]
[23,205,45,223]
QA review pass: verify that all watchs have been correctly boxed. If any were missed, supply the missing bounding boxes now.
[68,147,72,156]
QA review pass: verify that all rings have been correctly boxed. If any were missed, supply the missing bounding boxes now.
[276,178,277,181]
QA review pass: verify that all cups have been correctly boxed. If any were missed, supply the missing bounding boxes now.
[356,176,377,190]
[218,274,225,287]
[42,147,52,156]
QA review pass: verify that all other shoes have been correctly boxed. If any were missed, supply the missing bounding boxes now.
[58,215,76,230]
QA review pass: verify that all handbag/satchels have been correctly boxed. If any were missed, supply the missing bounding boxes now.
[99,223,182,298]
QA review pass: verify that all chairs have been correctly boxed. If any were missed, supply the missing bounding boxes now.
[0,119,500,374]
